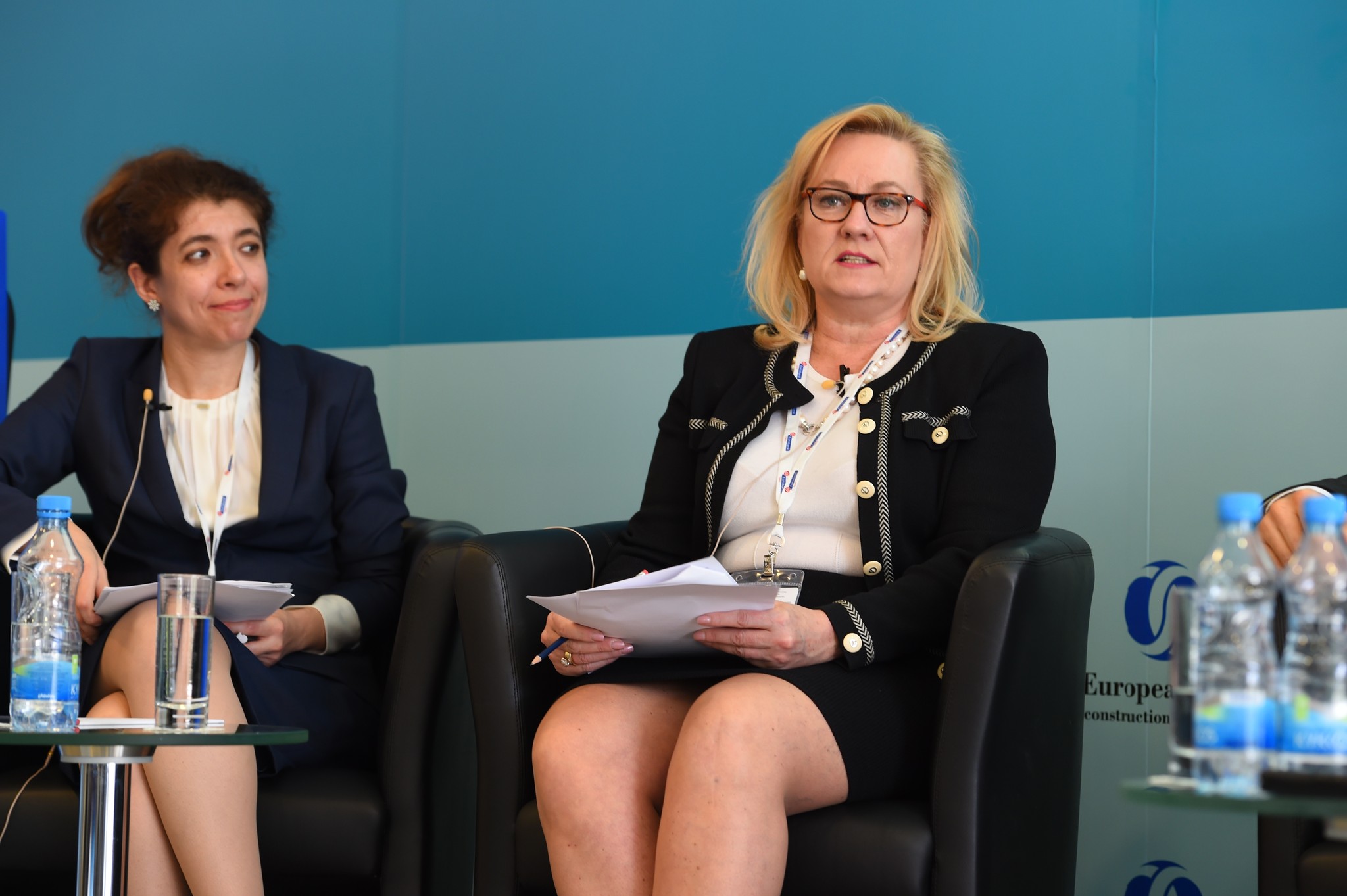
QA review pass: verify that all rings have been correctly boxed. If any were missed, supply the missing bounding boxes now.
[561,651,579,667]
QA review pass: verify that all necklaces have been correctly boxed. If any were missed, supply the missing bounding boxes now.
[791,323,911,436]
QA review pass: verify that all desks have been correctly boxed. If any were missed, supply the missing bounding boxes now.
[1114,775,1346,819]
[0,724,311,895]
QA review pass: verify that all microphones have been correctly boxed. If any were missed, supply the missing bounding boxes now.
[822,379,837,390]
[141,388,154,406]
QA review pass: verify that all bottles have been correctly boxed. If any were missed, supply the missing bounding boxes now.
[9,495,84,733]
[1190,492,1279,796]
[1264,495,1347,776]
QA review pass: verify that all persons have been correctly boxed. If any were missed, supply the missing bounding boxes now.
[534,105,1058,896]
[1250,475,1347,582]
[0,147,412,896]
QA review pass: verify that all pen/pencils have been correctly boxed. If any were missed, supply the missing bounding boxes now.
[529,568,649,667]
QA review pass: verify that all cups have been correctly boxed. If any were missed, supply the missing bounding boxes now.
[1167,587,1194,779]
[154,573,216,731]
[10,571,70,650]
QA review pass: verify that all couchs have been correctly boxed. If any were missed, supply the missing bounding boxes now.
[452,521,1097,896]
[0,512,484,896]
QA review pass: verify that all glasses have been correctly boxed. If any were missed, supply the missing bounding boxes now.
[800,186,931,226]
